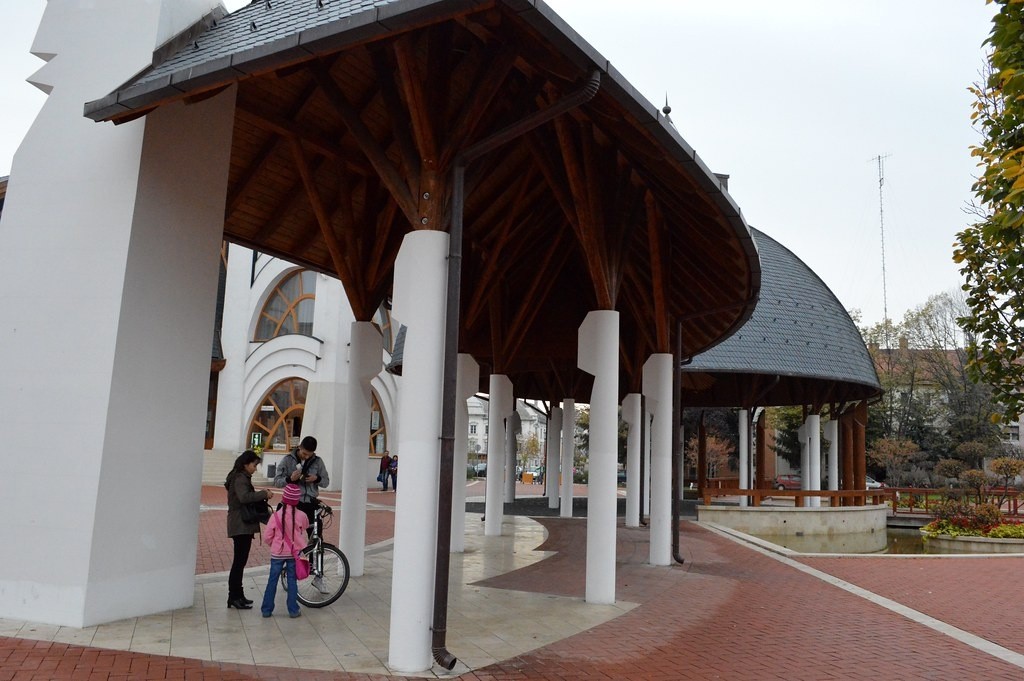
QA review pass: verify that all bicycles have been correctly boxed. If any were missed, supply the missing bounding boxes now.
[278,497,350,608]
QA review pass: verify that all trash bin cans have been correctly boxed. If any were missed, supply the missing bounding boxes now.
[267,464,276,478]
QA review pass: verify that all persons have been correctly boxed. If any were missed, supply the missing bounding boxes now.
[380,451,391,492]
[272,436,329,594]
[262,484,309,618]
[389,455,398,493]
[224,450,273,610]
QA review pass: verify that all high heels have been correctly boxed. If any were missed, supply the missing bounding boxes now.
[227,600,253,609]
[240,594,253,604]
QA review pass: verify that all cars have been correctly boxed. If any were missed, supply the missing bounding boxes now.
[474,463,487,476]
[573,468,585,483]
[775,474,802,490]
[516,466,540,479]
[864,476,889,488]
[618,470,627,484]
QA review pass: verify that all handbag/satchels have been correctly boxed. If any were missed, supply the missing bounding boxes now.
[296,557,310,580]
[391,467,397,475]
[377,474,382,481]
[241,500,269,522]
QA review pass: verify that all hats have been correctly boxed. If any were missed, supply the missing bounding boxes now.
[282,484,301,505]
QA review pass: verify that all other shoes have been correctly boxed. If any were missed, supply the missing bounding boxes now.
[289,610,301,617]
[392,490,395,492]
[263,611,271,617]
[381,489,387,491]
